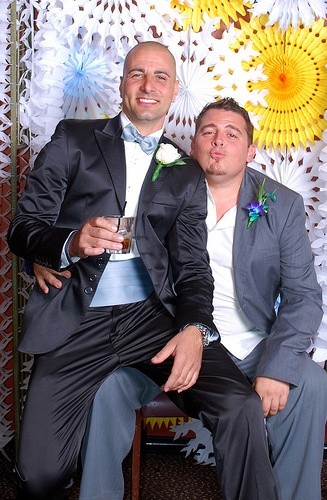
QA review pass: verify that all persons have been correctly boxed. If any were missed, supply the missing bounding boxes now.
[33,98,327,500]
[8,41,281,500]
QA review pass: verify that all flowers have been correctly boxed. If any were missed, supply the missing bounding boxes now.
[243,177,277,228]
[151,143,191,183]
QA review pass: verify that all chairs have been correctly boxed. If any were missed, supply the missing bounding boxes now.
[132,394,214,500]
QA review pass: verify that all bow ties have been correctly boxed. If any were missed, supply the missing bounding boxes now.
[120,123,161,155]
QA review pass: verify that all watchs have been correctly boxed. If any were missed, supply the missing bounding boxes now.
[195,323,209,347]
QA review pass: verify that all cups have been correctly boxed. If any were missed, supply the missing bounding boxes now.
[103,215,135,255]
